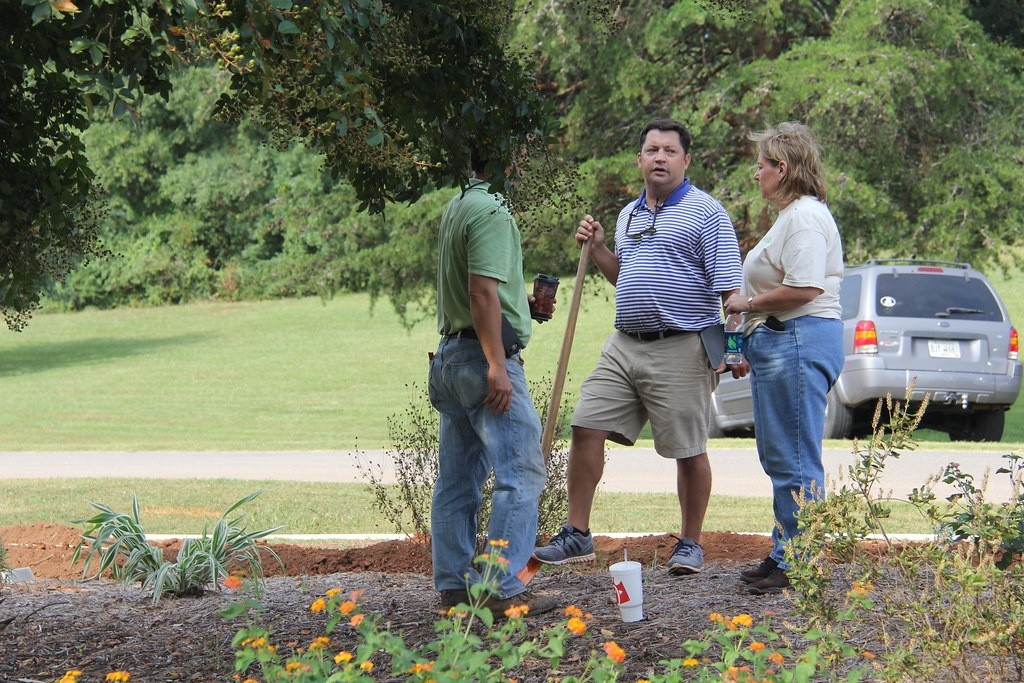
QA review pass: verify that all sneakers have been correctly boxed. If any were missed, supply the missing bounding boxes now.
[531,524,597,566]
[666,534,705,575]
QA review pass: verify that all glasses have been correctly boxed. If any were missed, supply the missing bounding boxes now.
[623,194,662,239]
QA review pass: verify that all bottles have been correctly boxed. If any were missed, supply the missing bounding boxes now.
[723,313,744,364]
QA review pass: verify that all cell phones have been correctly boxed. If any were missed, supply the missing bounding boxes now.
[765,315,785,331]
[500,314,523,357]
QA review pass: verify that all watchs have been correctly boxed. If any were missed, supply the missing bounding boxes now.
[747,295,754,313]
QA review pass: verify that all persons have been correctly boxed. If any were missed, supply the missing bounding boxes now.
[724,121,844,594]
[532,119,749,574]
[427,132,558,622]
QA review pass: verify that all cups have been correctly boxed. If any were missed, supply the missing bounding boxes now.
[530,273,560,321]
[609,561,643,623]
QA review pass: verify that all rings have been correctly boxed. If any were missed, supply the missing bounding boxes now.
[723,303,727,309]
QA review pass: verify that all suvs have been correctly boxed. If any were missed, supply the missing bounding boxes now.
[707,257,1024,441]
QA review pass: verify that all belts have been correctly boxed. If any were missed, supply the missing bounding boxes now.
[624,328,689,342]
[449,328,479,339]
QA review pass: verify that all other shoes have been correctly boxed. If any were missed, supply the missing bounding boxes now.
[440,588,471,612]
[479,589,564,624]
[748,567,793,595]
[740,557,780,583]
